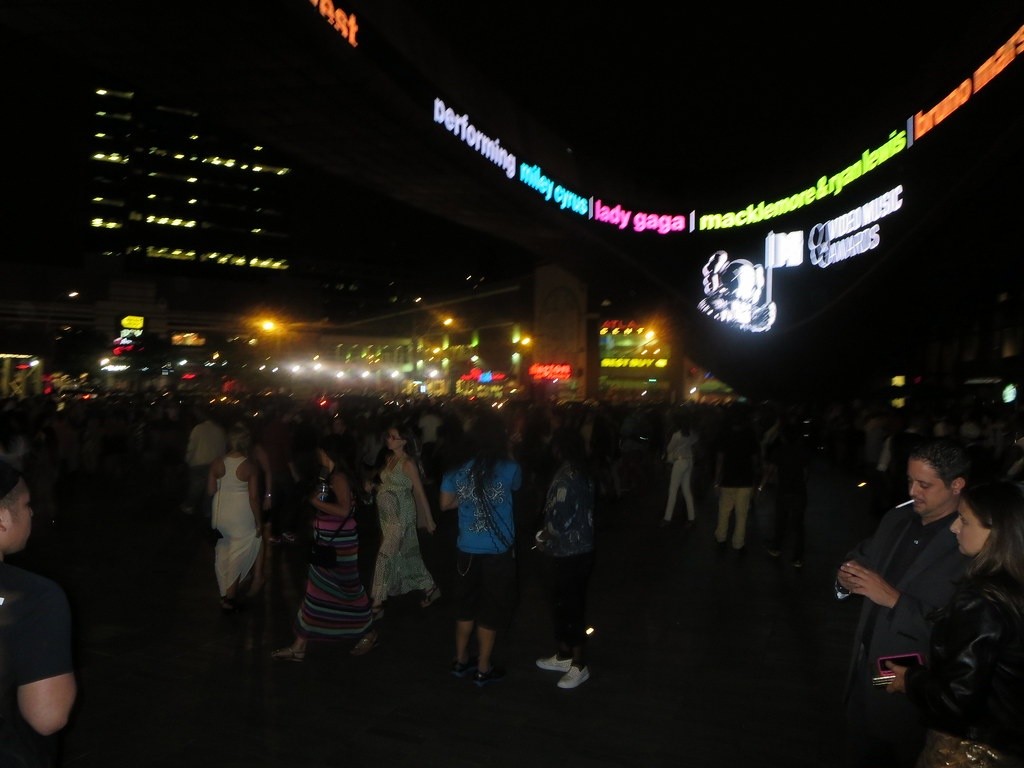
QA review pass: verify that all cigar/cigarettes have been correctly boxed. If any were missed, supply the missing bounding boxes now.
[895,499,915,509]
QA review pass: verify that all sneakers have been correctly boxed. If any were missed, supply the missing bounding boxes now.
[556,664,590,688]
[536,654,571,672]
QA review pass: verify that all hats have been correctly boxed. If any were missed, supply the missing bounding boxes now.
[0,460,19,499]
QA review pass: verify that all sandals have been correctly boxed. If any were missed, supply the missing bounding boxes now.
[273,648,306,663]
[350,631,380,656]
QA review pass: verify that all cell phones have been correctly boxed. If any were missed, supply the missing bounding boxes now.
[878,653,923,677]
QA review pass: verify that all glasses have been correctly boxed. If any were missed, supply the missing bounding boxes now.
[387,433,404,440]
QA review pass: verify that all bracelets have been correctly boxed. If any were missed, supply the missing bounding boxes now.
[833,579,851,600]
[535,529,545,544]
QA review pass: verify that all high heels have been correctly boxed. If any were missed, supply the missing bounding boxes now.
[370,604,385,620]
[421,588,440,606]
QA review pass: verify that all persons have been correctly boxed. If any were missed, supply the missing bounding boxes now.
[368,424,440,623]
[440,417,524,687]
[268,434,380,663]
[0,458,79,768]
[832,436,1024,768]
[531,427,606,690]
[0,387,1024,623]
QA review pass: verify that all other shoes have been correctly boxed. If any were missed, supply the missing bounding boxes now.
[659,520,672,529]
[791,559,802,567]
[473,666,506,686]
[685,521,695,529]
[452,656,475,677]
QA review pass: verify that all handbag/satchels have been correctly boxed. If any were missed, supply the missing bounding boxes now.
[304,539,338,569]
